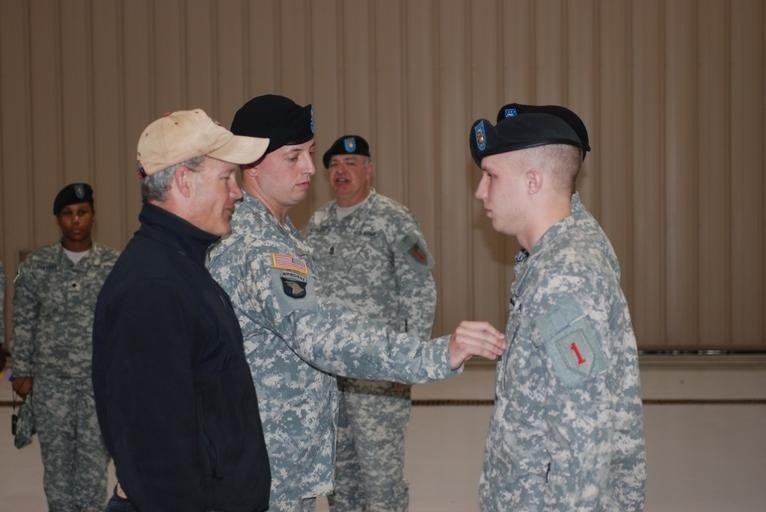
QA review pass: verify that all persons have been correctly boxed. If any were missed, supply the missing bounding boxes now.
[7,181,123,511]
[202,92,507,511]
[496,101,624,284]
[302,133,439,511]
[89,108,273,510]
[468,111,650,510]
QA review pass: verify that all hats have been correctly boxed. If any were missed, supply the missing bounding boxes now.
[470,103,591,167]
[137,108,270,176]
[231,94,313,152]
[54,183,92,214]
[323,135,369,169]
